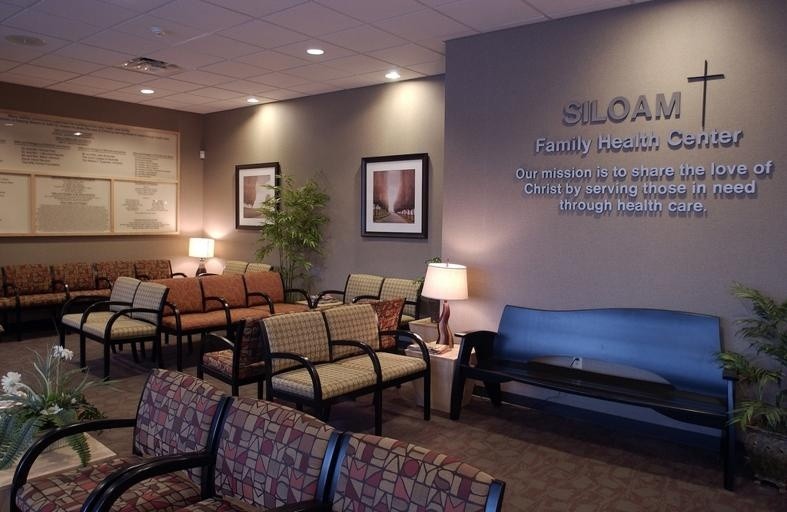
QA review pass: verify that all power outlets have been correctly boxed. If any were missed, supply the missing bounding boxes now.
[571,356,584,370]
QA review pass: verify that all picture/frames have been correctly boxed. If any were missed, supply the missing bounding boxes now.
[234,161,280,231]
[360,153,429,240]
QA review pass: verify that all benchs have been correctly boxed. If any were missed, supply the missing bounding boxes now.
[449,304,742,495]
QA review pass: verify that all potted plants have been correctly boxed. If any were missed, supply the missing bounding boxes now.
[712,280,787,496]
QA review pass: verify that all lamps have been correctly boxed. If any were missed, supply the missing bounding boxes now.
[188,237,216,278]
